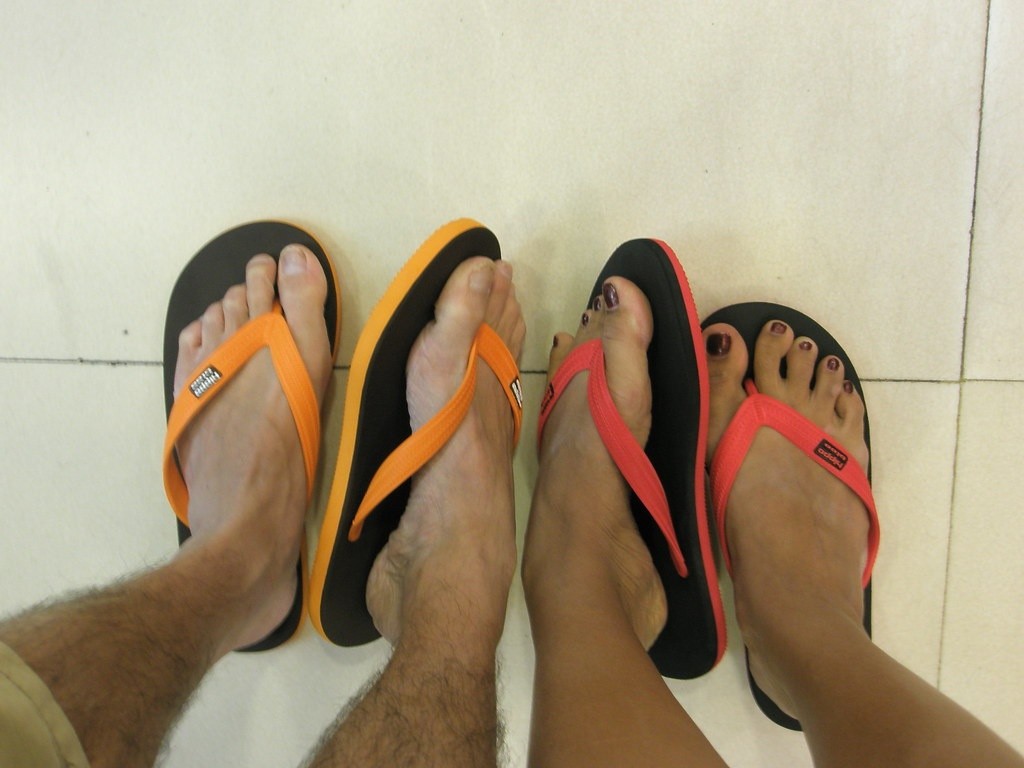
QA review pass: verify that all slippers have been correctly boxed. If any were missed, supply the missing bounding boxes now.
[162,219,342,652]
[700,302,880,731]
[537,237,728,681]
[308,218,524,647]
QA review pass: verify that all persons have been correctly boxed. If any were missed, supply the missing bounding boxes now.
[520,237,1024,768]
[0,213,525,768]
